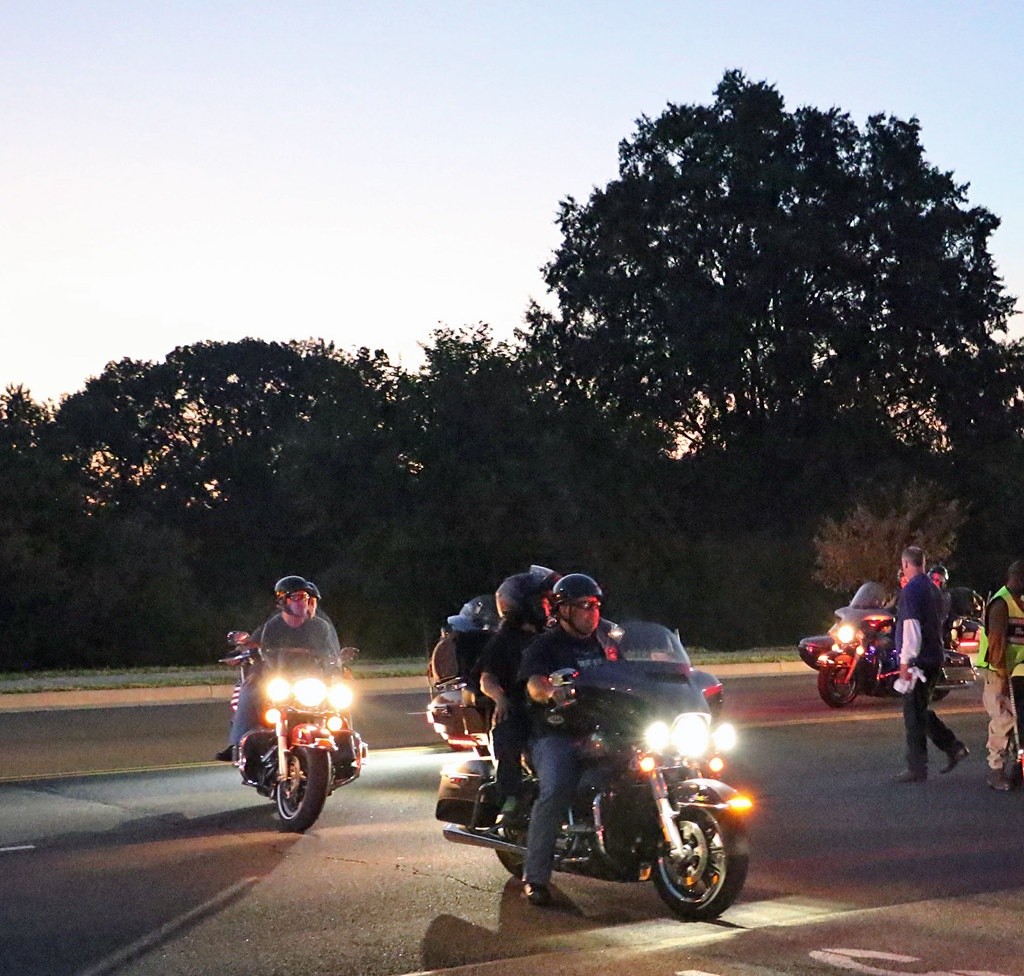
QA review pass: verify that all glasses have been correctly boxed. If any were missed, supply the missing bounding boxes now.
[577,600,601,610]
[289,594,309,601]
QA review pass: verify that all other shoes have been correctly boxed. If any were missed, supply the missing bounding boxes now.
[523,883,551,906]
[939,740,971,774]
[501,799,519,813]
[890,770,927,783]
[216,746,239,762]
[985,769,1015,791]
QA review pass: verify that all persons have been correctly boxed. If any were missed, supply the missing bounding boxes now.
[895,567,909,590]
[214,578,369,761]
[473,566,630,906]
[976,557,1024,791]
[889,545,971,783]
[926,564,949,591]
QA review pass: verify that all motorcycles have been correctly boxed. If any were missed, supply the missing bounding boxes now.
[797,582,980,708]
[425,620,750,921]
[218,630,362,833]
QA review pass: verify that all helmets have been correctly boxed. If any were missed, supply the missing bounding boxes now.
[552,573,605,611]
[495,565,563,619]
[306,582,322,601]
[274,576,313,601]
[929,565,948,581]
